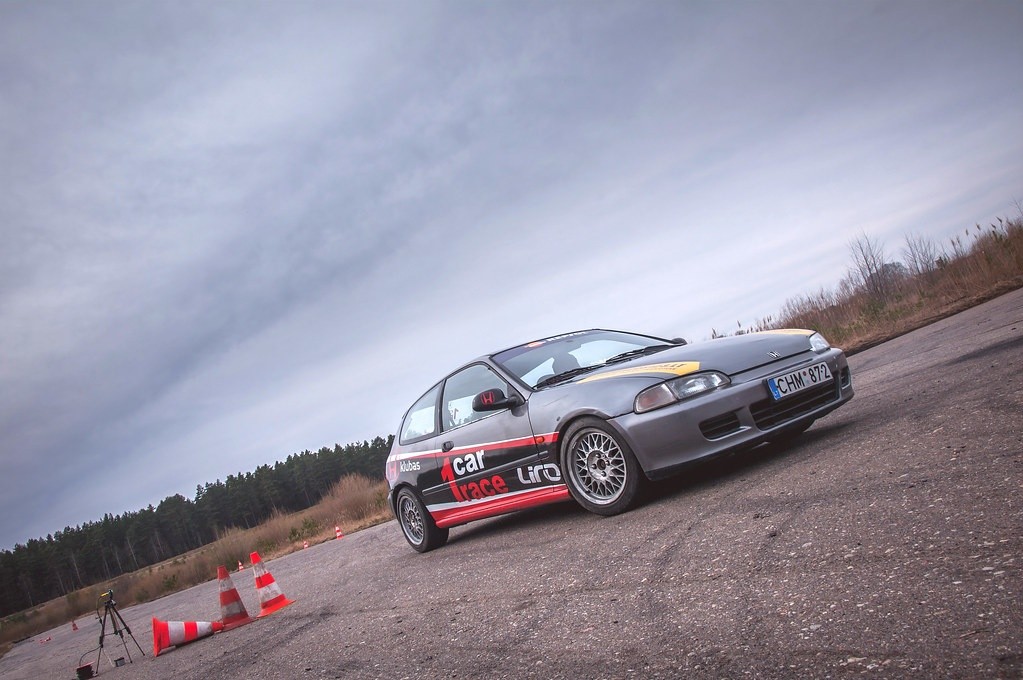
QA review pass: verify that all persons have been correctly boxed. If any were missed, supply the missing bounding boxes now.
[552,353,580,375]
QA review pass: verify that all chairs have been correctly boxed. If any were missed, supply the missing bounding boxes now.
[536,374,561,389]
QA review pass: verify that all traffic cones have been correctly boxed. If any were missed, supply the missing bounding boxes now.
[72,619,79,631]
[249,550,296,619]
[303,540,309,549]
[44,636,51,642]
[335,524,345,540]
[152,615,223,658]
[237,560,244,572]
[215,566,258,635]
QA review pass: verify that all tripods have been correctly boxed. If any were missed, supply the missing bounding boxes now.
[94,589,145,674]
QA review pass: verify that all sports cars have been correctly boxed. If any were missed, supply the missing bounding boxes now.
[384,326,855,556]
